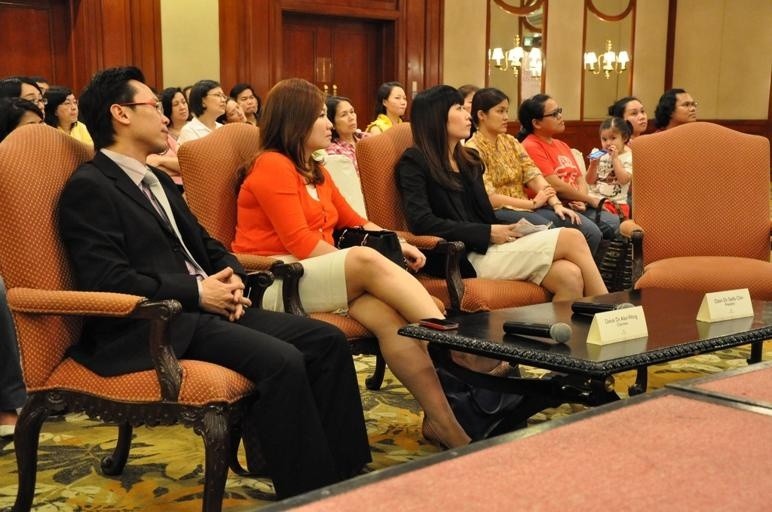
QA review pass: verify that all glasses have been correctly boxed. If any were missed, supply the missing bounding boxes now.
[544,108,562,117]
[27,98,48,105]
[119,102,164,114]
[208,93,227,101]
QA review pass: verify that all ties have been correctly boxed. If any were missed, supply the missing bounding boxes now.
[143,170,208,279]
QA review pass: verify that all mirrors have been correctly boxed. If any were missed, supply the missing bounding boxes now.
[580,0,637,122]
[485,0,548,122]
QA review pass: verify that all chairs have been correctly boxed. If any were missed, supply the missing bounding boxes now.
[0,123,255,512]
[631,121,772,302]
[177,122,446,389]
[355,122,552,312]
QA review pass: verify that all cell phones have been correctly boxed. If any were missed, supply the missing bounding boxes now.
[418,317,460,331]
[586,148,610,161]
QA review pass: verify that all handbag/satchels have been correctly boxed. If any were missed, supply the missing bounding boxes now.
[334,226,406,269]
[431,368,528,442]
[596,198,633,292]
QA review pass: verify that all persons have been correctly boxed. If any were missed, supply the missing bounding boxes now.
[1,78,406,200]
[58,66,373,499]
[232,77,474,451]
[396,86,608,302]
[0,276,27,441]
[459,81,697,257]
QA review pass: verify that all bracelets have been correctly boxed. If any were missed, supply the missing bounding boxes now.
[400,238,406,243]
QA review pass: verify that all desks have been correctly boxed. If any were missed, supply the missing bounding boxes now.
[397,288,772,445]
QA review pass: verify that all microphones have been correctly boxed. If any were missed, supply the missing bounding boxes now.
[502,320,573,344]
[571,301,636,315]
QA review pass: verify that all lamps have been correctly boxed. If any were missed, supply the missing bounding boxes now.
[584,39,630,79]
[492,34,542,78]
